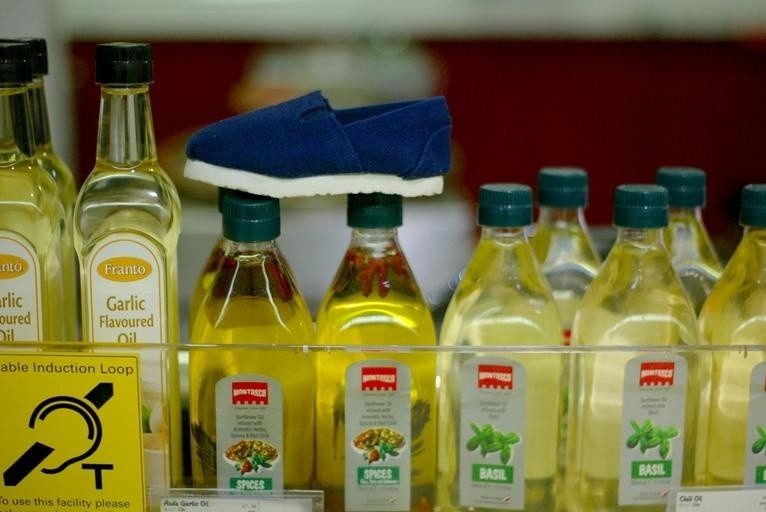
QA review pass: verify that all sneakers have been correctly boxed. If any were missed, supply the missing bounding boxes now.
[183,90,454,199]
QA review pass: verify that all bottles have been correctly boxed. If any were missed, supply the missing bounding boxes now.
[567,183,714,511]
[437,185,567,512]
[185,186,236,333]
[71,40,181,512]
[0,38,80,354]
[530,167,601,512]
[654,166,725,326]
[315,192,437,511]
[0,39,65,351]
[694,183,766,490]
[187,197,315,490]
